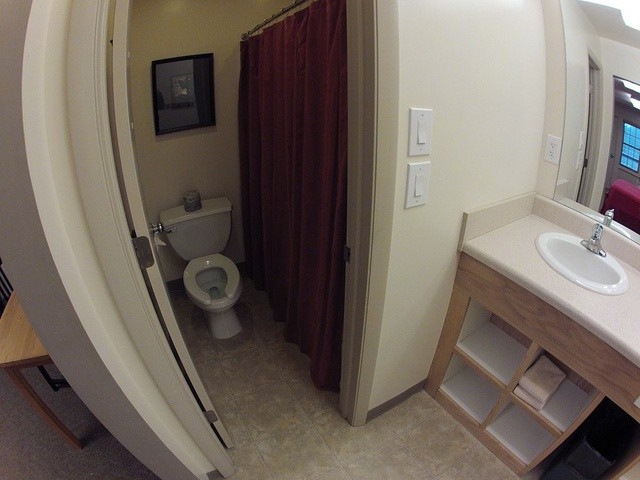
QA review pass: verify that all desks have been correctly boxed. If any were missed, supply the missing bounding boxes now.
[0,289,83,449]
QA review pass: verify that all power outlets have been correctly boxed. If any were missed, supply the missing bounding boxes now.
[543,134,562,165]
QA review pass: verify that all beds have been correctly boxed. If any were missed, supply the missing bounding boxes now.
[602,179,640,234]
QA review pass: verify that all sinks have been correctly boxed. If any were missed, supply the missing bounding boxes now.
[535,232,629,295]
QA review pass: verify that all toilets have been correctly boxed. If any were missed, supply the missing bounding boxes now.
[159,196,243,339]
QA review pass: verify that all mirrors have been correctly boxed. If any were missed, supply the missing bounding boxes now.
[553,18,640,245]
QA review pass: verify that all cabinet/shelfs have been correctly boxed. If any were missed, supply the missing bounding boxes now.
[423,252,639,480]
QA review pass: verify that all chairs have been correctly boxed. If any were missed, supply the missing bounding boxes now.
[0,266,72,391]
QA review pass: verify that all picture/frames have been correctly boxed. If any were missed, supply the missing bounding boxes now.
[151,53,216,136]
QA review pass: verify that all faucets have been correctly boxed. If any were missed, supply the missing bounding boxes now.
[580,223,607,258]
[603,208,615,227]
